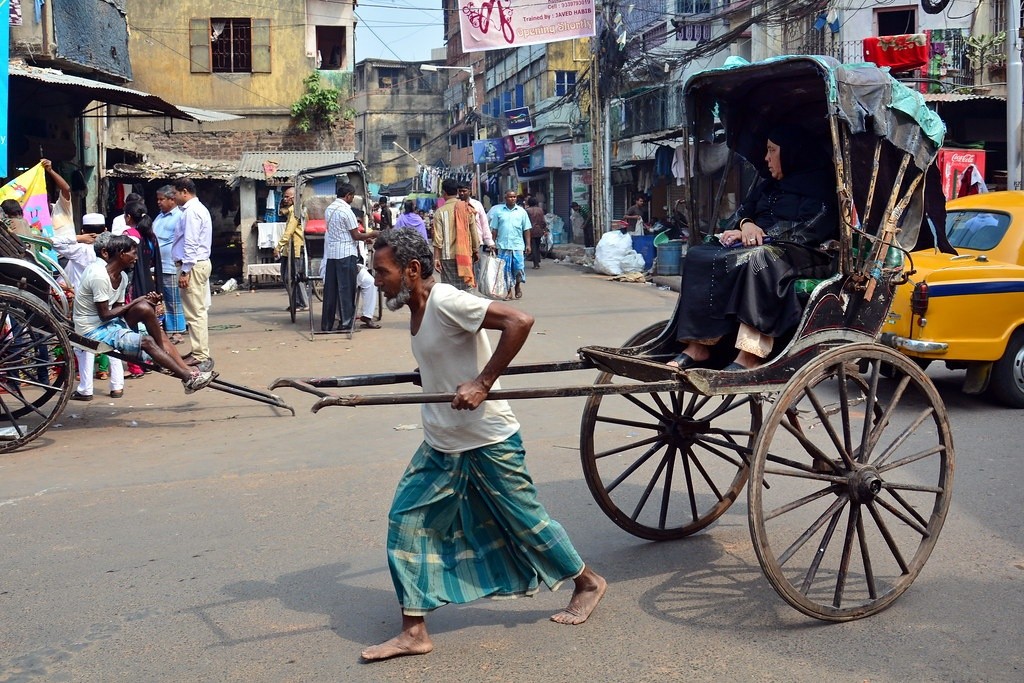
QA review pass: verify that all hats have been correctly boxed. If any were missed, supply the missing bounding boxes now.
[82,213,105,225]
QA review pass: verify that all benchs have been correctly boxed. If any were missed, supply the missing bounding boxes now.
[303,196,365,236]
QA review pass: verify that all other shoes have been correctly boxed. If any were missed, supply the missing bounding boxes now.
[182,370,219,394]
[183,356,201,366]
[124,373,144,379]
[0,383,17,394]
[77,374,80,380]
[287,305,309,311]
[181,352,192,360]
[532,266,538,269]
[168,337,185,344]
[96,370,108,380]
[195,357,214,372]
[143,367,151,373]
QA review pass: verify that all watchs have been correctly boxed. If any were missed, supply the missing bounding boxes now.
[181,270,187,276]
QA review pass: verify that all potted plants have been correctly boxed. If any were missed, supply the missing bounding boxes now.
[966,30,1006,94]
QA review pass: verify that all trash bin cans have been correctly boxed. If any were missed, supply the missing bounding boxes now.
[657,243,681,275]
[631,235,654,270]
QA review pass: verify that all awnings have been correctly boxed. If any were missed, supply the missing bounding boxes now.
[7,65,246,121]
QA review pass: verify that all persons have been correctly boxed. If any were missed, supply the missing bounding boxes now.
[372,178,547,302]
[319,182,381,331]
[489,143,498,158]
[73,230,221,394]
[0,199,57,387]
[274,187,309,312]
[665,108,839,372]
[171,178,212,366]
[121,201,164,380]
[151,185,186,346]
[50,213,124,401]
[318,208,382,329]
[40,158,78,270]
[622,196,644,232]
[361,227,608,660]
[570,202,594,248]
[111,193,145,236]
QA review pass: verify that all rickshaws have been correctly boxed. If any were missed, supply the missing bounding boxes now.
[265,54,957,623]
[285,161,383,325]
[0,209,295,453]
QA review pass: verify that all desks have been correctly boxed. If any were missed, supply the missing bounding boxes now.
[247,263,281,292]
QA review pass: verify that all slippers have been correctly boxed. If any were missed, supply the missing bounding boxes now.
[360,324,381,329]
[722,362,747,372]
[70,391,92,401]
[111,389,123,398]
[667,351,706,369]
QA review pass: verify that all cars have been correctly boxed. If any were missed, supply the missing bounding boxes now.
[872,188,1023,407]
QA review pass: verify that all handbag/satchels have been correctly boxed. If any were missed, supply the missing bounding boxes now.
[539,243,547,253]
[478,250,508,299]
